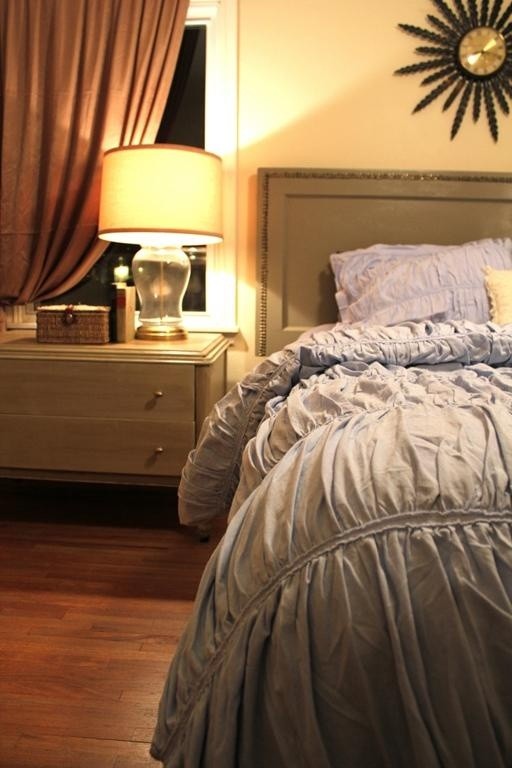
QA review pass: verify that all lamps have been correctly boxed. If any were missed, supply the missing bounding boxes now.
[98,144,225,341]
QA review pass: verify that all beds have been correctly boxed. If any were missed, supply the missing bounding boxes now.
[148,168,511,768]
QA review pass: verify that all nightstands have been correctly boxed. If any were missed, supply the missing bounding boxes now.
[0,331,235,544]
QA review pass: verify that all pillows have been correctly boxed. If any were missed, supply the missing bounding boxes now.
[329,238,511,324]
[482,263,512,325]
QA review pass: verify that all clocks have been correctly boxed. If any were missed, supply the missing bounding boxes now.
[394,0,512,142]
